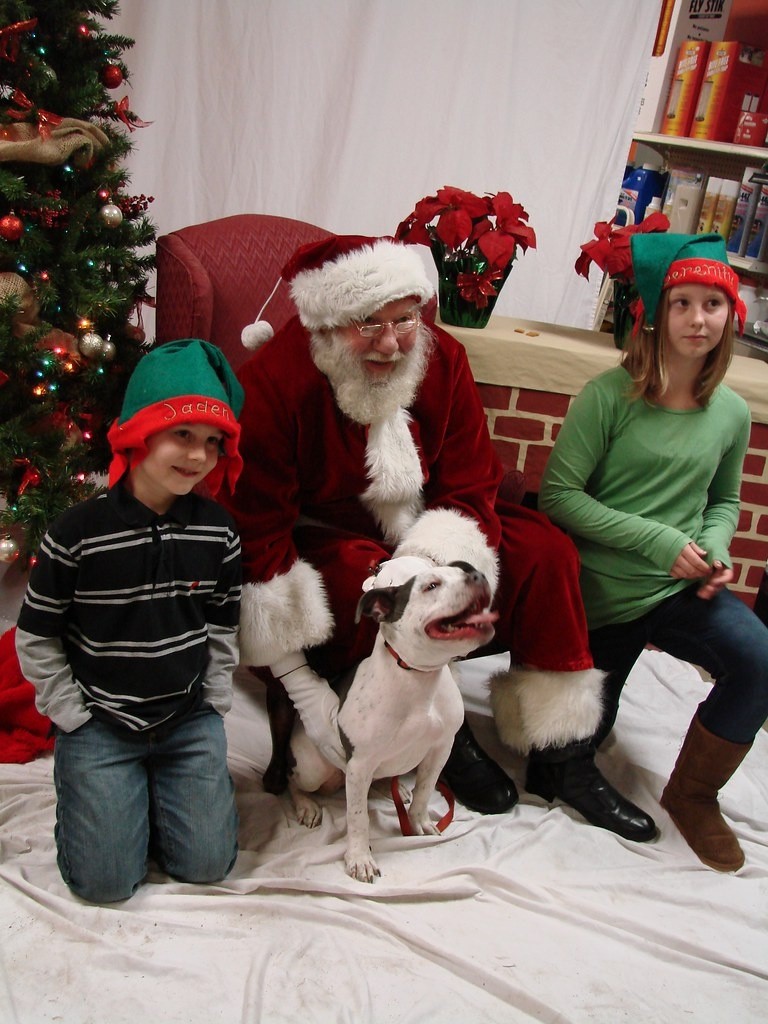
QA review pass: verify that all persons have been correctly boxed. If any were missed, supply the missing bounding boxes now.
[15,338,245,904]
[542,213,768,872]
[229,235,655,839]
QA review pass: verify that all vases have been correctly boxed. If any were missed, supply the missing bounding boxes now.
[438,252,515,330]
[612,282,643,351]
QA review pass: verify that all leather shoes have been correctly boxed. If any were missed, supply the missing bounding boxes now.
[525,740,657,841]
[444,715,519,816]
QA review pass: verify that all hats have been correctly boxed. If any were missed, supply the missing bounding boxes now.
[630,228,748,343]
[242,235,438,351]
[107,337,244,500]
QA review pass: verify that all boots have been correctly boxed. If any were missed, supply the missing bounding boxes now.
[660,702,755,871]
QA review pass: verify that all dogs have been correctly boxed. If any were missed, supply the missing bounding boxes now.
[263,560,502,886]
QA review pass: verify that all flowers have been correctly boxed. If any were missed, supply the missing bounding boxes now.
[392,183,537,308]
[574,210,671,340]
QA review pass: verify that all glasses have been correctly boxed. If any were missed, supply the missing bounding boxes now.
[348,312,419,337]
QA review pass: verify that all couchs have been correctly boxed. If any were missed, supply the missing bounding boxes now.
[155,214,334,371]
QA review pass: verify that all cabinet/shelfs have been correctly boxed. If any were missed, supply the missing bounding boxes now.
[588,0,768,361]
